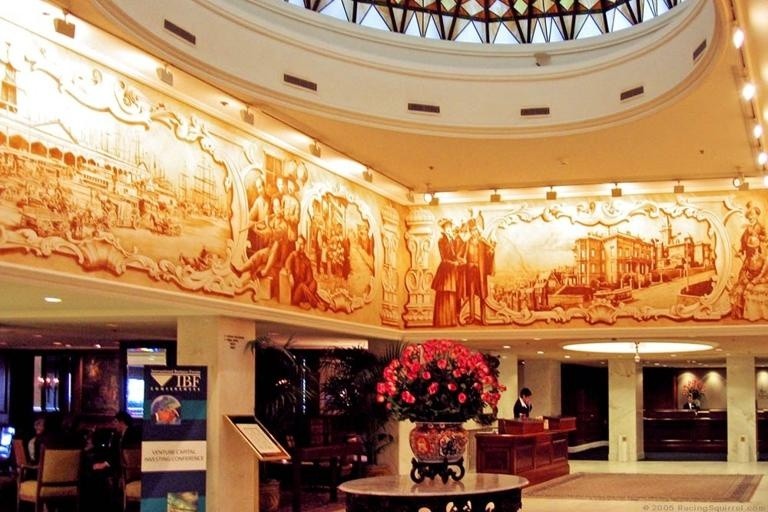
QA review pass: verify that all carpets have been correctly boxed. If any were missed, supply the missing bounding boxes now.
[519,469,765,503]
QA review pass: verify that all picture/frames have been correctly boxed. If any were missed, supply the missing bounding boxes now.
[219,414,291,464]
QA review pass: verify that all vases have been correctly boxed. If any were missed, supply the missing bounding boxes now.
[409,420,467,464]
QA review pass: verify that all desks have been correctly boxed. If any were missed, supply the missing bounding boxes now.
[336,473,531,511]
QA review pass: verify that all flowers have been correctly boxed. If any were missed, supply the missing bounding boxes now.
[373,337,508,427]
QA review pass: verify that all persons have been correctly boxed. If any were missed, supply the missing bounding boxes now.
[28,416,46,461]
[514,390,533,419]
[45,416,88,484]
[683,395,696,409]
[91,410,138,480]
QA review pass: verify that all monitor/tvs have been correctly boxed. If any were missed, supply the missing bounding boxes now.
[0,423,18,463]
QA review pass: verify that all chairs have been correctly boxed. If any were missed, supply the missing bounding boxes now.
[10,438,85,511]
